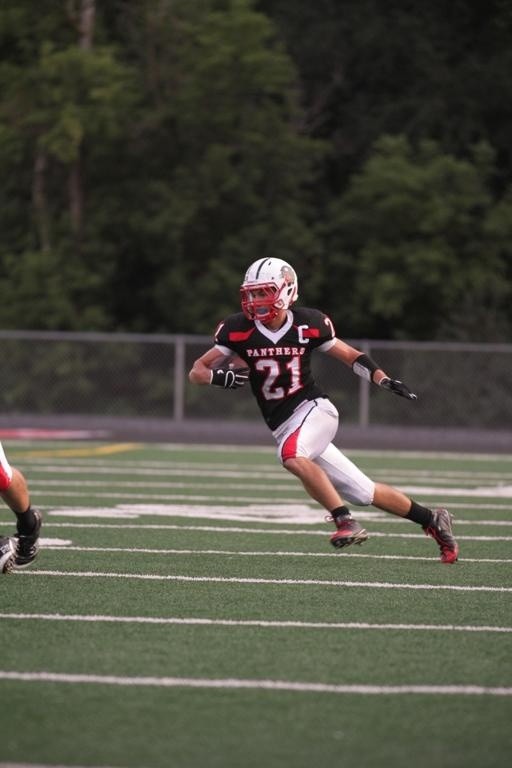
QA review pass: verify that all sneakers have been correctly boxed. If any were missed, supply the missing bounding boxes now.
[421,509,457,562]
[14,509,42,567]
[331,519,369,547]
[0,535,20,574]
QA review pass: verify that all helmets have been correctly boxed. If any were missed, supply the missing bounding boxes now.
[239,257,298,321]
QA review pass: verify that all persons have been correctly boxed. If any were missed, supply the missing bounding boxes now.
[2,442,42,578]
[185,256,461,563]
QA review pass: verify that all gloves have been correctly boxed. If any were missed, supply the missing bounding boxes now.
[210,362,250,390]
[379,375,417,400]
